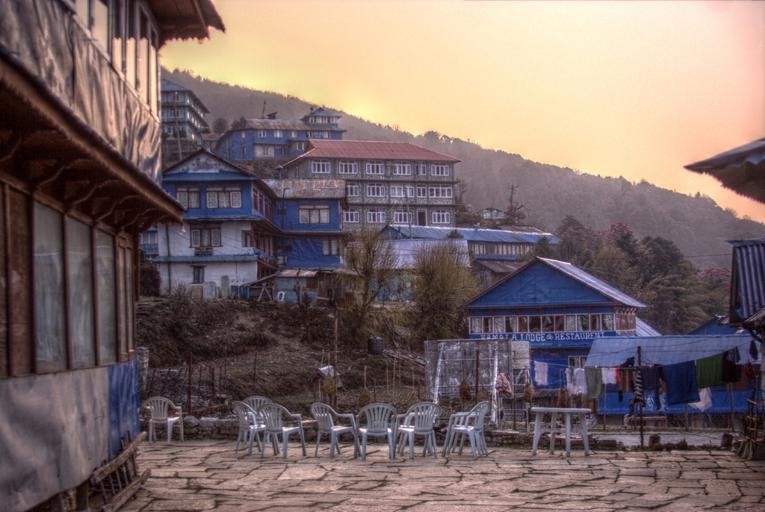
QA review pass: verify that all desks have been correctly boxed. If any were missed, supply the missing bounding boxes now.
[531,407,592,457]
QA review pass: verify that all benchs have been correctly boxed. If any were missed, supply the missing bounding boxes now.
[631,415,668,429]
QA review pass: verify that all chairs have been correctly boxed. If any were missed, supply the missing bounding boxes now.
[144,397,185,443]
[231,395,490,459]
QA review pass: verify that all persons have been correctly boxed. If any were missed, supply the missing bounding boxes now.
[623,398,640,426]
[658,375,669,413]
